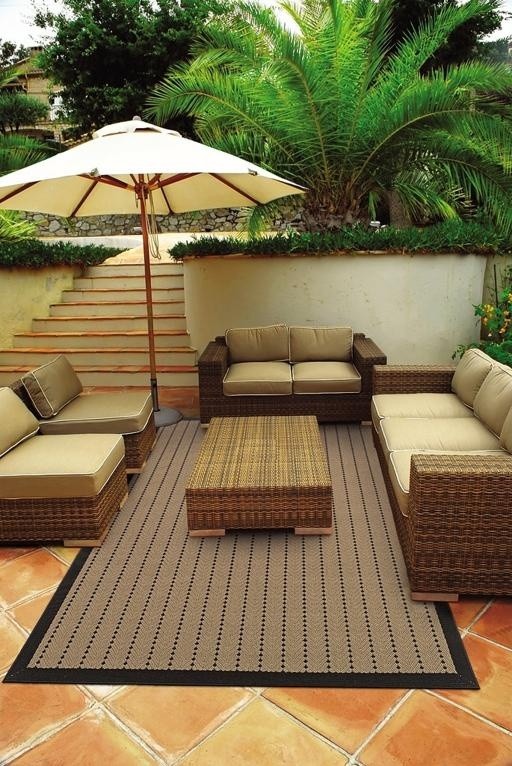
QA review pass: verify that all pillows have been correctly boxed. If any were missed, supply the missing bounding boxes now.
[0,386,40,458]
[224,323,353,362]
[21,354,83,419]
[451,348,512,456]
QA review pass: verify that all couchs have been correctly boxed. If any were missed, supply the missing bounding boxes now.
[371,348,512,602]
[198,324,387,426]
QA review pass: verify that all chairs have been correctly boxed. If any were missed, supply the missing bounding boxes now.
[0,387,129,547]
[10,354,157,474]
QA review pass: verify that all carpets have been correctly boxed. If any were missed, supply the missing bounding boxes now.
[2,415,481,690]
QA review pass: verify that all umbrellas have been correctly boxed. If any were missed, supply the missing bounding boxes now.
[0,114,309,414]
